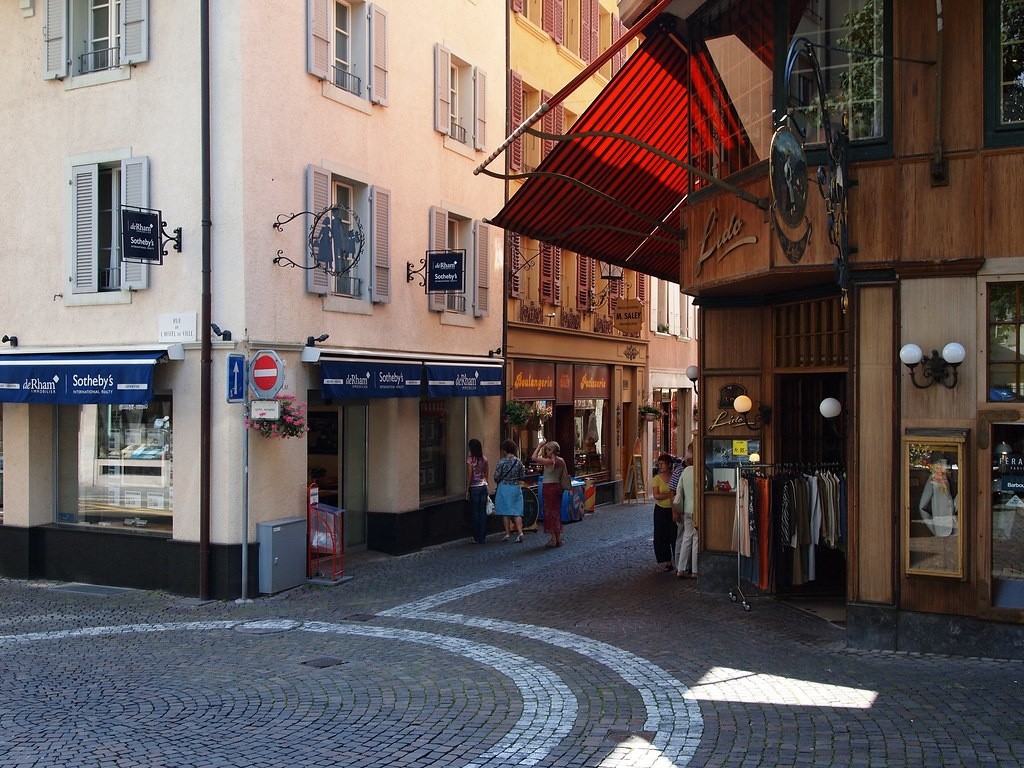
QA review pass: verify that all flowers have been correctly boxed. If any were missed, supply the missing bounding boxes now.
[525,405,554,423]
[243,394,305,444]
[503,396,530,432]
[639,402,668,417]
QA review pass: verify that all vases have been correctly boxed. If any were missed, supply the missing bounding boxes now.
[524,413,539,431]
[646,413,655,421]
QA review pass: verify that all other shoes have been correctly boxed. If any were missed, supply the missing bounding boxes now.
[467,538,480,544]
[691,573,698,579]
[676,573,684,577]
[545,539,562,546]
[664,563,673,572]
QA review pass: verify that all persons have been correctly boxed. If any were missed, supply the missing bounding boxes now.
[531,440,567,547]
[465,439,489,544]
[784,154,796,215]
[493,440,525,543]
[651,454,698,577]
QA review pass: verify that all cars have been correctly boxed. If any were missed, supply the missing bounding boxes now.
[108,443,173,460]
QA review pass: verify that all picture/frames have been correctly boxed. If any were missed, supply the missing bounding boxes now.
[901,432,970,581]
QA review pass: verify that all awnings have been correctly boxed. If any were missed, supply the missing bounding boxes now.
[482,18,761,283]
[0,350,167,404]
[300,346,504,400]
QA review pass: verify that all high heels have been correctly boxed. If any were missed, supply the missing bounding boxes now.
[515,536,524,544]
[500,535,509,544]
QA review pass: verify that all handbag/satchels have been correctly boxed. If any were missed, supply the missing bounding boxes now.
[672,486,683,516]
[560,471,572,490]
[486,496,496,516]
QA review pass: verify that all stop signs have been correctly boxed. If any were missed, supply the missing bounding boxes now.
[248,349,285,398]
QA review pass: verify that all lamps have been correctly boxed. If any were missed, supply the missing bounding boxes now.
[212,324,232,340]
[687,363,699,396]
[489,348,502,358]
[898,340,965,390]
[306,334,329,347]
[590,259,634,310]
[819,399,860,419]
[546,313,556,326]
[735,395,770,435]
[2,335,18,346]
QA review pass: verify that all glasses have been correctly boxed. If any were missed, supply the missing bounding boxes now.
[658,462,668,465]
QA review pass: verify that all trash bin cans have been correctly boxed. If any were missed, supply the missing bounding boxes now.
[584,477,596,513]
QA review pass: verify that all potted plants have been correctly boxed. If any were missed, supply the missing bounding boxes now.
[306,466,326,484]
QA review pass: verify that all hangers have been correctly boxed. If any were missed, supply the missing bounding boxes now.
[740,463,845,479]
